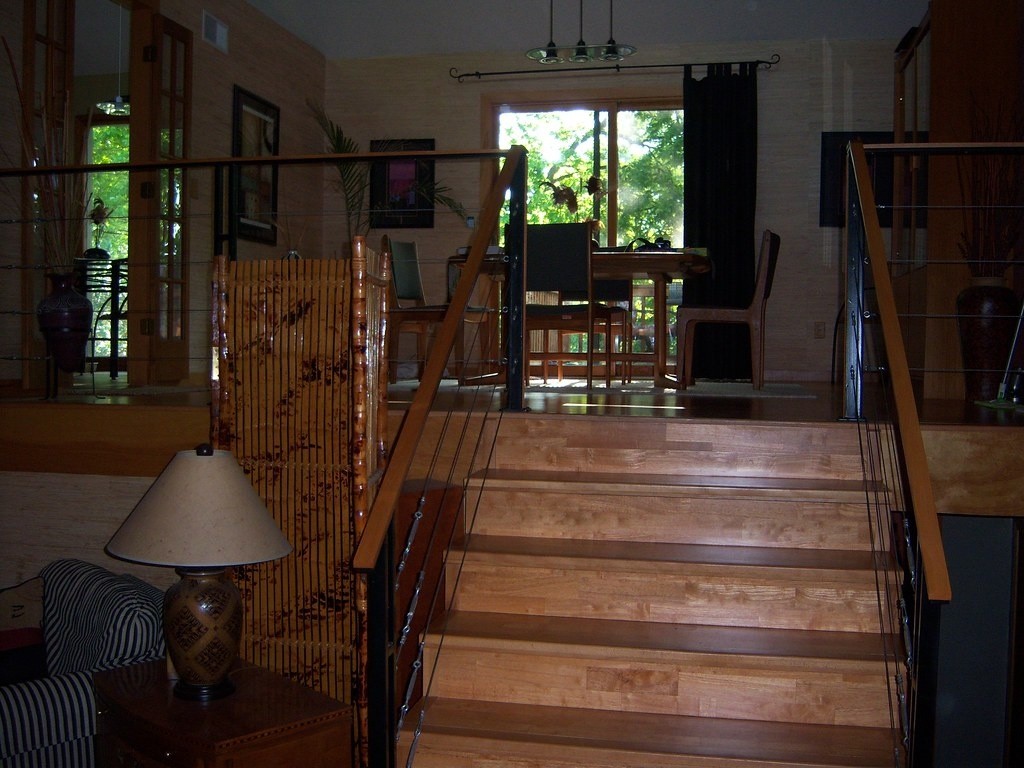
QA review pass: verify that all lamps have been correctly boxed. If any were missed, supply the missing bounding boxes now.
[104,444,294,705]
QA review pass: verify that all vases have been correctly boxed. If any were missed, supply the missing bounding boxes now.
[281,250,303,279]
[955,276,1024,424]
[35,272,93,369]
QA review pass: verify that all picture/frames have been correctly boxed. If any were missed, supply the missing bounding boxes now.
[367,138,437,228]
[230,82,280,245]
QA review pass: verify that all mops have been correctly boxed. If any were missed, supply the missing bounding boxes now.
[973,302,1024,409]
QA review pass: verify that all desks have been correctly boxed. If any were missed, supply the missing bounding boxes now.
[449,251,712,388]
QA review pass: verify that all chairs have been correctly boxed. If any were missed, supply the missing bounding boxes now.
[675,229,782,391]
[382,234,495,388]
[556,243,636,383]
[0,558,166,768]
[502,222,611,390]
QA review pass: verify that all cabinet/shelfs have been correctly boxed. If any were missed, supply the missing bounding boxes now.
[93,654,355,768]
